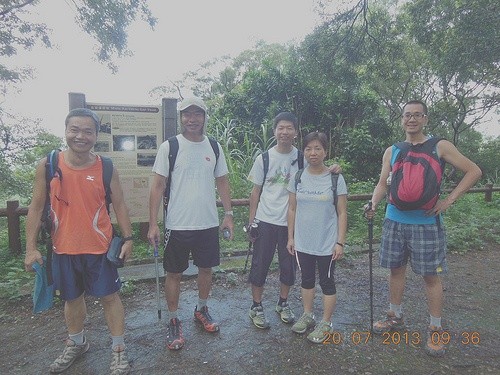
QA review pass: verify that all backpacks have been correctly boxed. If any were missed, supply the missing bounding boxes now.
[387,137,447,210]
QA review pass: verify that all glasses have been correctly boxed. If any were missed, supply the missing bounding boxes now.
[402,112,426,121]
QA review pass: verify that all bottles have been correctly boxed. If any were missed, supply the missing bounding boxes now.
[387,172,392,200]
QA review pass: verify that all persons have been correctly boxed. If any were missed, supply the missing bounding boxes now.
[364,101,481,357]
[283,133,348,343]
[247,112,342,329]
[147,97,234,350]
[25,108,133,375]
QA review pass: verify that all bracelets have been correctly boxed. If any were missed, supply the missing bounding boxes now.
[336,242,344,247]
[124,236,133,241]
[224,210,234,216]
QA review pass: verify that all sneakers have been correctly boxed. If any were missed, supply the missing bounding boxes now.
[372,310,405,335]
[165,317,185,350]
[110,343,130,375]
[194,305,219,333]
[291,312,316,333]
[307,320,333,343]
[248,303,271,329]
[274,300,295,323]
[425,324,448,356]
[49,335,90,373]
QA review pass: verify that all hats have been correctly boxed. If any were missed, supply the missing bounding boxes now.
[178,97,209,130]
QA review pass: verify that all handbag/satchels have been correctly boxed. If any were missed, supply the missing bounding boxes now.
[32,256,55,314]
